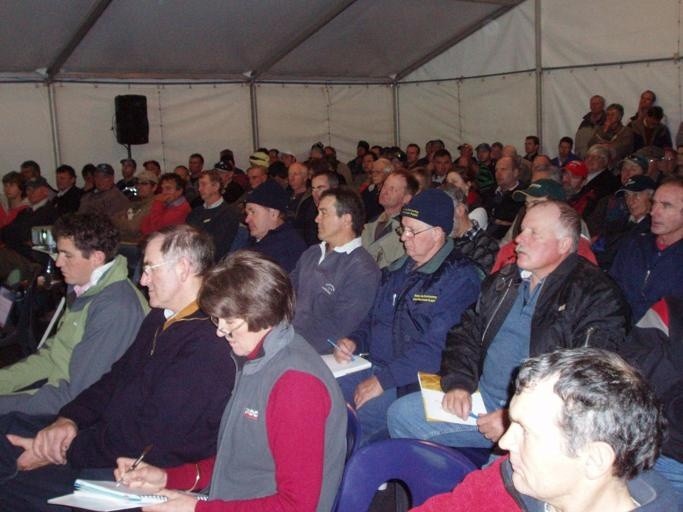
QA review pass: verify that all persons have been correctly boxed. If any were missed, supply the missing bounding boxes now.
[0,91,683,512]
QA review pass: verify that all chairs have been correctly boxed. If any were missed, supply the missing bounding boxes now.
[331,400,480,511]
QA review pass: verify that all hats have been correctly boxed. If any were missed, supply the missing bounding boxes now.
[214,161,234,173]
[512,178,567,206]
[23,163,115,189]
[274,137,408,162]
[457,143,473,152]
[244,179,291,214]
[616,155,650,170]
[132,170,158,186]
[399,187,455,236]
[474,143,492,153]
[615,175,656,198]
[560,159,589,181]
[249,151,270,169]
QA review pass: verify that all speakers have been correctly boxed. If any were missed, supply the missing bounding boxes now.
[114,94,149,144]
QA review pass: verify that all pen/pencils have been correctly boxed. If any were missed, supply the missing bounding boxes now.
[327,339,356,360]
[115,445,153,488]
[469,413,479,419]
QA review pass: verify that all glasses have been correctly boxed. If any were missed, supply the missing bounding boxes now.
[141,258,178,274]
[211,315,247,339]
[395,226,439,239]
